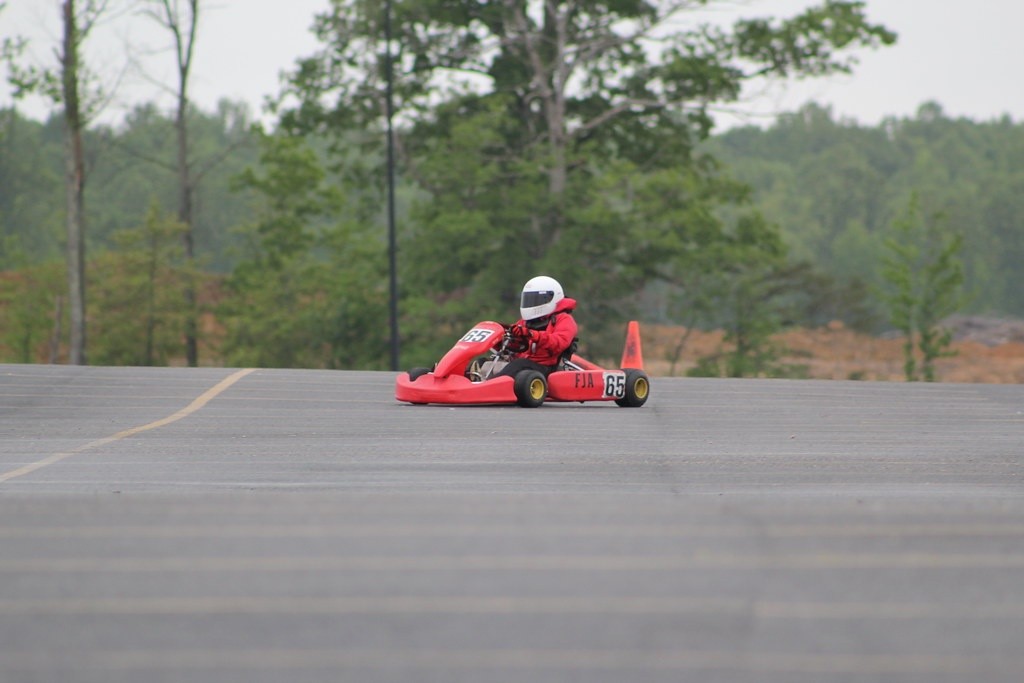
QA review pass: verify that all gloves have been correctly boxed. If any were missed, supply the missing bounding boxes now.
[509,324,529,337]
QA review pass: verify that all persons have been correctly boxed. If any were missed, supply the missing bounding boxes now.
[429,275,578,383]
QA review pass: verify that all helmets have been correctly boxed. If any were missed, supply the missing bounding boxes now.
[520,275,565,320]
